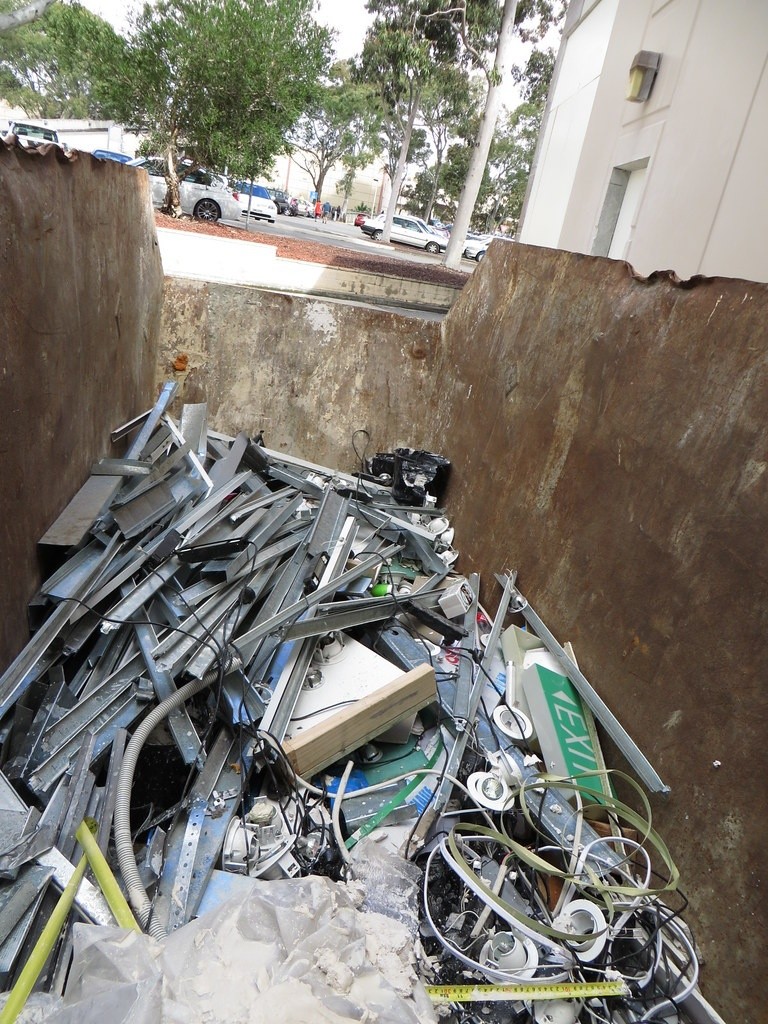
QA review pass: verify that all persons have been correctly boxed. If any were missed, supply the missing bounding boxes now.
[290,197,341,224]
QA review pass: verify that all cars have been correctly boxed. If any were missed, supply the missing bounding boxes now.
[264,187,317,219]
[91,148,132,168]
[234,179,277,225]
[3,119,64,149]
[431,223,515,262]
[354,212,371,225]
[361,214,448,255]
[124,157,243,225]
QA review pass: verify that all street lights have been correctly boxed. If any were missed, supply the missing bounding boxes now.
[370,178,380,221]
